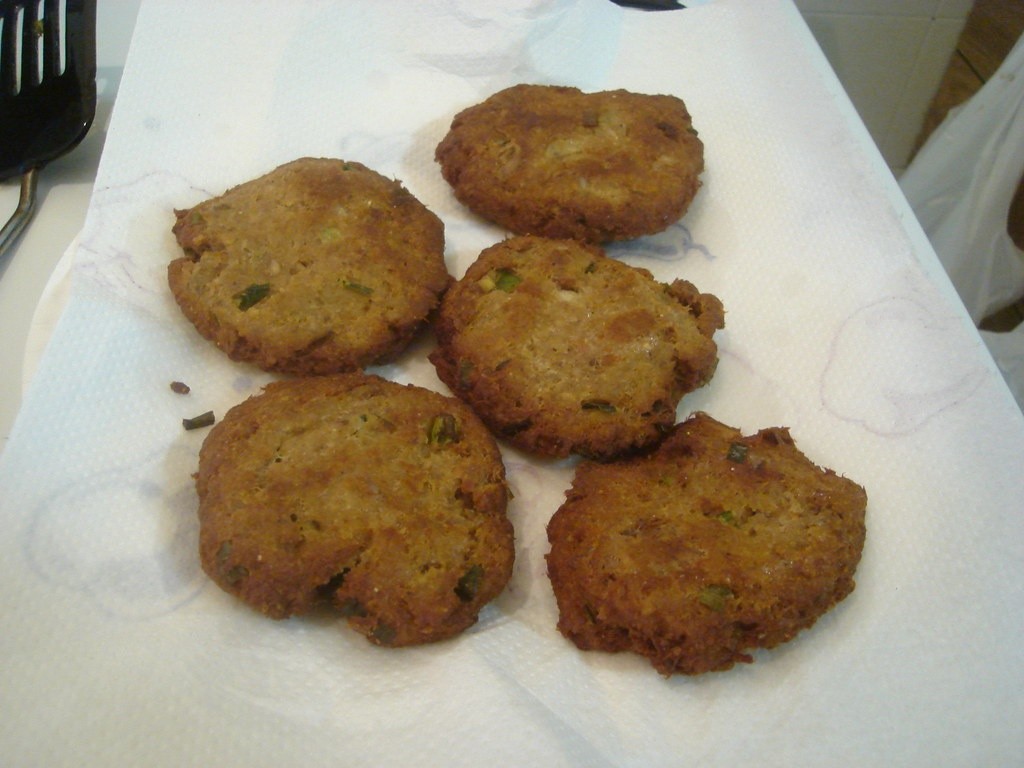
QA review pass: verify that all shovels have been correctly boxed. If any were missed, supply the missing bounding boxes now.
[0,0,100,254]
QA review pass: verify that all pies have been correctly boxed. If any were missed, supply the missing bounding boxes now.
[167,83,868,676]
